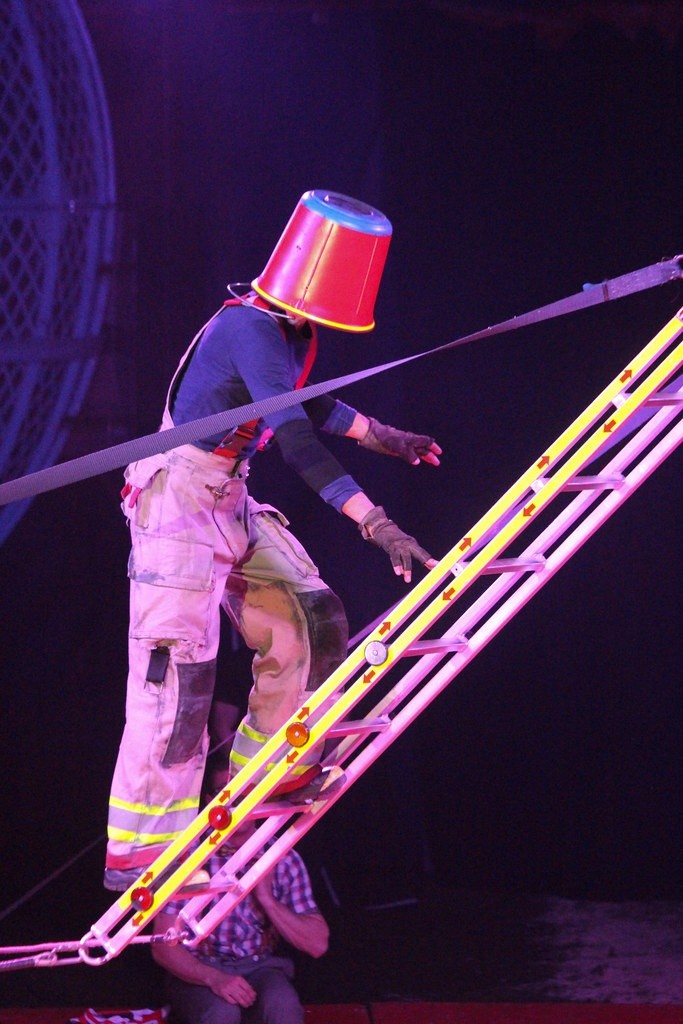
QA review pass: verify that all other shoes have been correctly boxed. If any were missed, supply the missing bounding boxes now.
[233,765,348,805]
[103,860,210,892]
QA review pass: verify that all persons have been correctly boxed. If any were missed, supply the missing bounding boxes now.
[100,190,443,890]
[149,759,330,1024]
[210,642,258,761]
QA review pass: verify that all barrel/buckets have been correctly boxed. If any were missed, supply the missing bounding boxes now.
[226,189,394,333]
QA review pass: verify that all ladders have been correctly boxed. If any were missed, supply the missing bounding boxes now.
[77,303,683,965]
